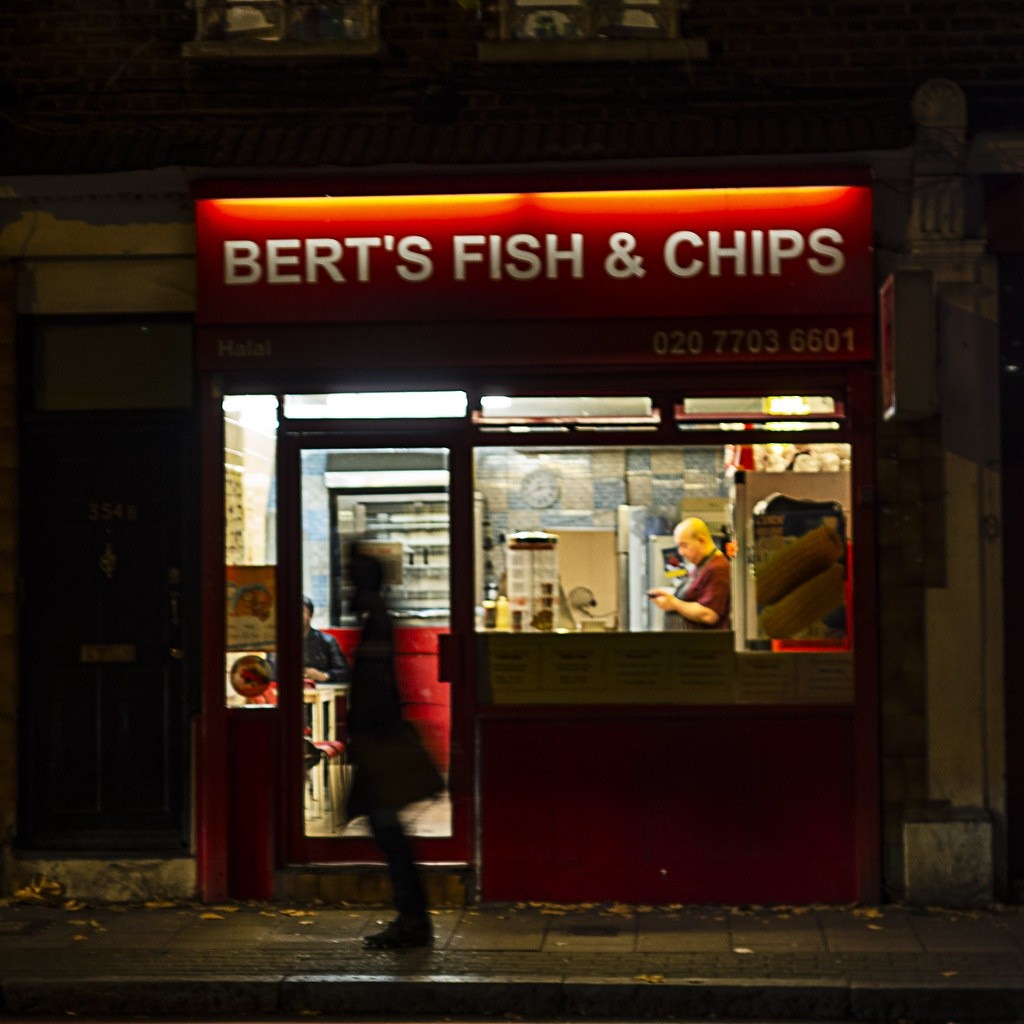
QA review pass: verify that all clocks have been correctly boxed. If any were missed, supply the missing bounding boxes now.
[518,469,561,510]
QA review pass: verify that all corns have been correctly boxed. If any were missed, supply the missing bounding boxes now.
[753,524,847,635]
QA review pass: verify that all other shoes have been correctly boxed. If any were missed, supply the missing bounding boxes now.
[363,918,434,949]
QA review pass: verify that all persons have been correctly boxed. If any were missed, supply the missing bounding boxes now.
[336,546,448,950]
[644,517,731,629]
[266,594,349,684]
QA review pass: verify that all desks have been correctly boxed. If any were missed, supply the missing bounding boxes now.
[303,683,347,818]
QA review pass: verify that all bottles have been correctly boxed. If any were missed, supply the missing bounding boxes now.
[495,596,509,628]
